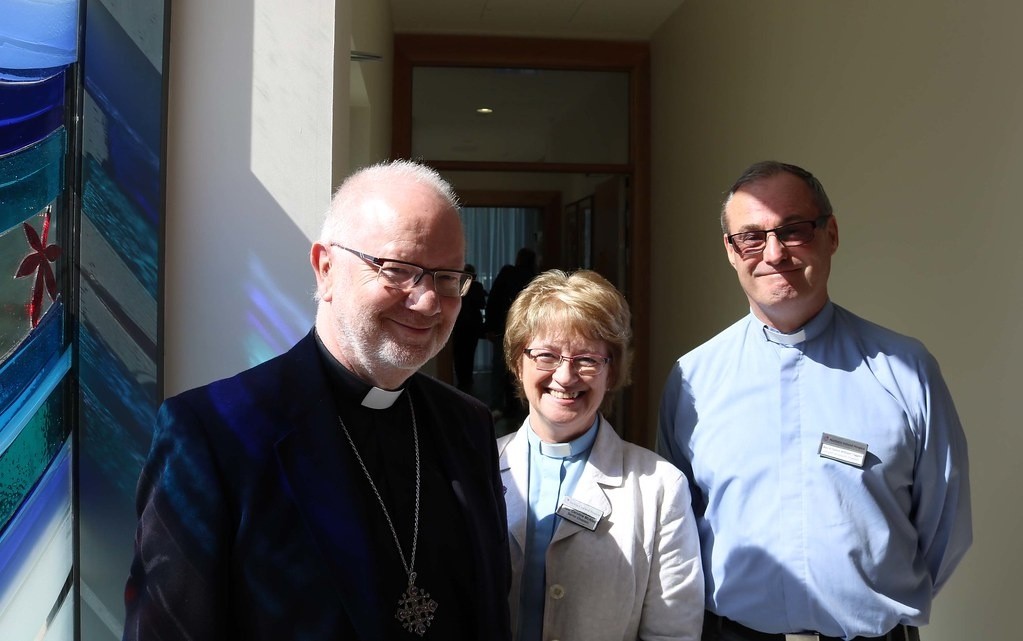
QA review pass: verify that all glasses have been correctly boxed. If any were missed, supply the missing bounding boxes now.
[330,243,477,297]
[728,216,827,255]
[523,348,611,375]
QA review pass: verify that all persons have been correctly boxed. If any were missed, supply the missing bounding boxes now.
[443,240,572,420]
[123,160,512,641]
[655,161,972,641]
[495,269,705,641]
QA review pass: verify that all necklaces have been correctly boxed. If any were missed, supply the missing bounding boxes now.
[337,386,439,636]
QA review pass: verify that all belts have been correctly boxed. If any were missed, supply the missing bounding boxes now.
[721,618,904,641]
